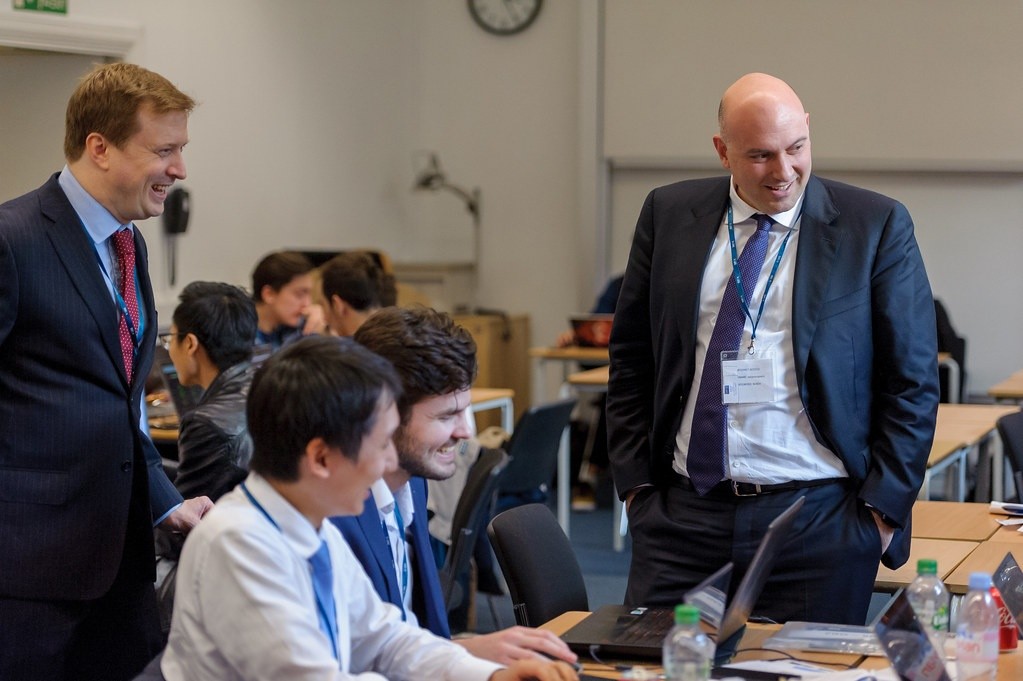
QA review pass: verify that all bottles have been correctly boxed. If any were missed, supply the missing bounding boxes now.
[876,623,951,681]
[906,559,950,671]
[662,605,712,681]
[955,572,999,681]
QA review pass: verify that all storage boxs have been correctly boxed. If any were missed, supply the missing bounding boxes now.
[398,285,531,434]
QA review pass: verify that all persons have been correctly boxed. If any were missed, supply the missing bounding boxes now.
[161,333,580,680]
[933,299,963,402]
[607,73,939,625]
[557,272,624,508]
[0,62,195,681]
[140,250,398,535]
[330,307,578,666]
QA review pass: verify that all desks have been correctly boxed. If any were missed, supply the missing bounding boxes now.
[148,344,1023,681]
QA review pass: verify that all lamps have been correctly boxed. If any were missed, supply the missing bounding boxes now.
[413,149,480,223]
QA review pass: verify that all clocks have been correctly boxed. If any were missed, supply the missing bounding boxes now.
[469,0,543,36]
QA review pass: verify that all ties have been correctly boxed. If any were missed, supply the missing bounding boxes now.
[686,212,780,499]
[308,540,341,669]
[110,226,138,384]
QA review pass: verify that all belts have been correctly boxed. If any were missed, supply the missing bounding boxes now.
[668,472,844,497]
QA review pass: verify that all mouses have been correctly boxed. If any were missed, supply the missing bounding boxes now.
[536,650,581,673]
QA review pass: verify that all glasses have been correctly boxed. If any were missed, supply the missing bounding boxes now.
[158,332,184,351]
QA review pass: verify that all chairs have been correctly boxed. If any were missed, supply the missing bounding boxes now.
[996,409,1023,504]
[442,448,509,620]
[475,397,579,594]
[487,503,590,628]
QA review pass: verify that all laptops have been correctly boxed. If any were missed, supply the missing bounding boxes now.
[157,361,206,417]
[557,495,807,661]
[990,552,1023,636]
[871,587,952,681]
